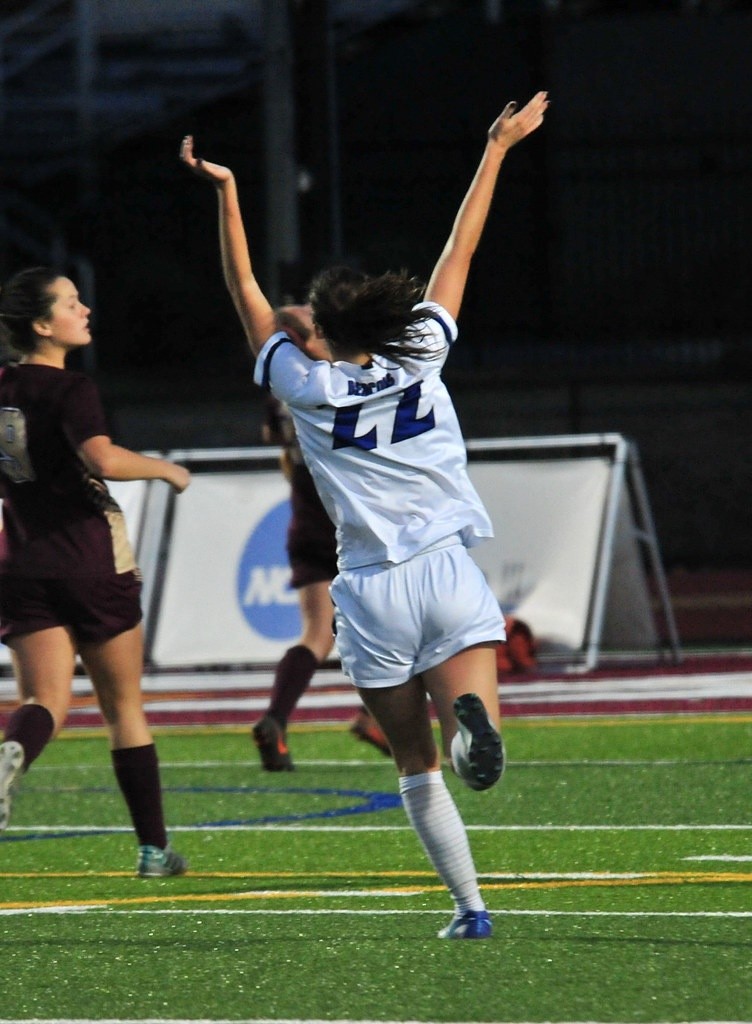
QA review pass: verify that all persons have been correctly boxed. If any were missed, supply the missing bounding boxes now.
[254,307,392,768]
[181,88,554,936]
[0,266,189,879]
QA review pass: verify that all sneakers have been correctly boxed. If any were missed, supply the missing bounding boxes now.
[0,740,26,831]
[437,909,492,939]
[453,693,504,785]
[253,716,295,771]
[137,844,188,878]
[349,707,392,757]
